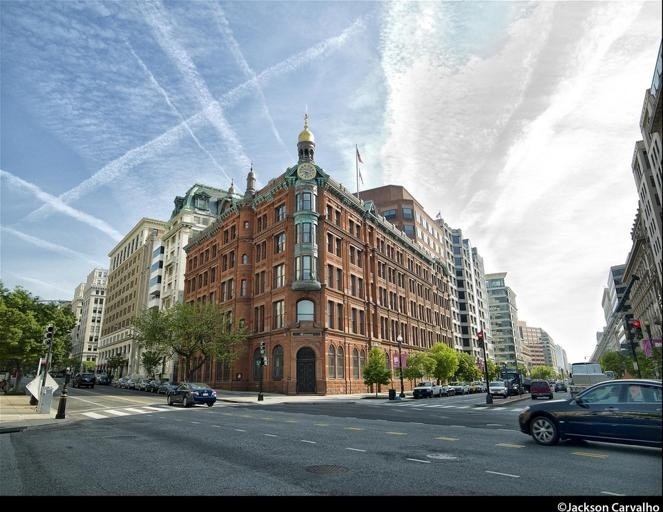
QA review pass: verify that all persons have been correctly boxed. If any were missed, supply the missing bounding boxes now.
[63,366,73,387]
[586,386,619,404]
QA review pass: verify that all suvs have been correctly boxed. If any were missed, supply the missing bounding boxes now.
[488,381,508,399]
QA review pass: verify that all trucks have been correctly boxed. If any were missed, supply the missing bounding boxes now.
[570,363,614,400]
[499,370,531,396]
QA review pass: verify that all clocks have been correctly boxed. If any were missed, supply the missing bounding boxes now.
[297,162,316,181]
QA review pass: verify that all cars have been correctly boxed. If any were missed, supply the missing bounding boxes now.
[529,380,553,400]
[555,382,567,392]
[95,373,177,395]
[72,371,95,389]
[412,378,487,399]
[166,382,216,408]
[517,377,662,448]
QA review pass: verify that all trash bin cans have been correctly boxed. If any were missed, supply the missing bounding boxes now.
[389,389,396,400]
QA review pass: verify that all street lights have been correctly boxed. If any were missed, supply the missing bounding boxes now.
[396,335,405,398]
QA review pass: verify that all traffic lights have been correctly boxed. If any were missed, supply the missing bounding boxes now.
[44,323,53,346]
[261,342,265,354]
[477,331,484,349]
[633,320,642,341]
